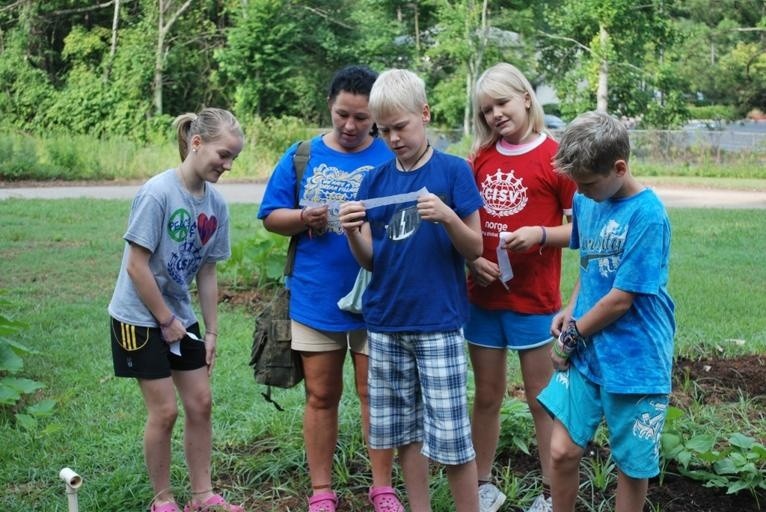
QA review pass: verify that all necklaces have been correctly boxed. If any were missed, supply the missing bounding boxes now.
[178,163,192,194]
[396,138,431,173]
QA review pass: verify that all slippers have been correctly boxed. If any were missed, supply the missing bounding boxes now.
[150,501,183,512]
[308,489,339,512]
[368,484,406,512]
[183,493,246,512]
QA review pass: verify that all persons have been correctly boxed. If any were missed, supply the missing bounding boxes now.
[457,61,577,512]
[536,111,677,511]
[107,106,247,512]
[336,68,486,512]
[256,63,407,512]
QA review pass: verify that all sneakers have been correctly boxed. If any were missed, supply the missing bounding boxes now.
[526,492,553,512]
[477,482,507,512]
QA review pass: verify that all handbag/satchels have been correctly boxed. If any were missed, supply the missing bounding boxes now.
[248,286,305,390]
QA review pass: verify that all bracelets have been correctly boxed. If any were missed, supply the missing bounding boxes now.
[553,319,586,359]
[158,313,176,329]
[537,224,547,247]
[300,205,312,229]
